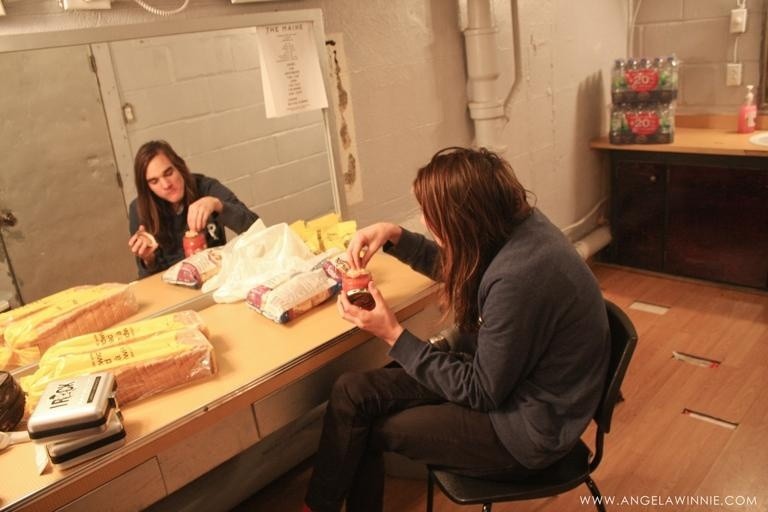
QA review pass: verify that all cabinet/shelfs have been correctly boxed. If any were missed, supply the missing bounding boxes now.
[2,237,447,511]
[608,150,768,291]
[2,263,220,377]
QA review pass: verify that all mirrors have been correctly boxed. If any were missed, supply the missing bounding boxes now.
[0,8,350,378]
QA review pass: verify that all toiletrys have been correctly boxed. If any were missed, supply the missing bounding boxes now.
[737,85,758,133]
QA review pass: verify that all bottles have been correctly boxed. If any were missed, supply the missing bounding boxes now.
[609,56,677,144]
[343,257,377,312]
[181,230,208,260]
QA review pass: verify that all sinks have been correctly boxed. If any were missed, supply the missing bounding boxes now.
[750,131,768,147]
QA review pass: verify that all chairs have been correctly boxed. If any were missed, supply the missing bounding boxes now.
[426,299,637,512]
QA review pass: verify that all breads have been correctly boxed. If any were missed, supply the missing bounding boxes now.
[110,310,217,409]
[1,281,140,366]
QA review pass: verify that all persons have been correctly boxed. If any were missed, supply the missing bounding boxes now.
[126,139,263,282]
[298,147,612,511]
